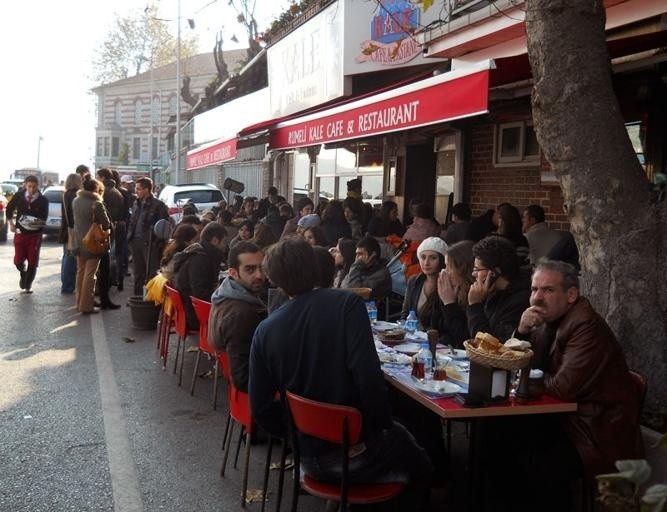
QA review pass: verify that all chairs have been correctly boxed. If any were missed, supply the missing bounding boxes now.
[572,370,647,512]
[156,267,177,360]
[165,283,198,386]
[189,295,222,410]
[284,387,404,512]
[259,386,292,511]
[217,349,251,477]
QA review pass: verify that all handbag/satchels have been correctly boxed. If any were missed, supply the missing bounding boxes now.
[82,204,109,255]
[62,194,78,257]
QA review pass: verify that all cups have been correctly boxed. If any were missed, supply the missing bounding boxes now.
[411,354,426,382]
[431,357,447,381]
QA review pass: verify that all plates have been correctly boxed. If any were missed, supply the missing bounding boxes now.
[419,379,461,394]
[393,344,423,353]
[451,360,470,371]
[374,320,396,330]
[373,339,393,354]
[406,330,427,342]
[379,353,411,364]
[380,340,406,346]
[436,348,467,358]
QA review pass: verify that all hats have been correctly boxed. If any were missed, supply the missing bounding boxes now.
[416,236,448,260]
[297,213,321,227]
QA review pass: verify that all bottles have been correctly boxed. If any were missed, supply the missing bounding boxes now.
[407,310,418,332]
[417,343,433,378]
[366,303,380,327]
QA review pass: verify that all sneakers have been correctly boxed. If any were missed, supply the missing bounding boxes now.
[82,307,100,314]
[95,301,101,306]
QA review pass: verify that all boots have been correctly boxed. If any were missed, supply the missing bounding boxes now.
[16,264,26,289]
[25,265,37,290]
[100,288,121,310]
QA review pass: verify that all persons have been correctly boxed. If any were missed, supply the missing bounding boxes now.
[262,246,336,319]
[316,198,327,218]
[277,202,294,217]
[372,203,383,219]
[76,165,90,185]
[475,260,645,512]
[237,197,259,225]
[333,239,356,289]
[426,241,477,350]
[231,195,242,214]
[71,174,111,317]
[466,200,511,242]
[109,170,130,292]
[315,199,352,249]
[362,202,383,235]
[214,211,239,244]
[95,168,124,295]
[252,223,277,248]
[170,221,227,303]
[246,242,454,512]
[212,200,226,214]
[468,235,533,341]
[401,201,442,242]
[278,197,315,242]
[119,174,137,276]
[133,177,168,297]
[343,197,367,246]
[180,214,202,235]
[400,237,448,332]
[491,206,530,264]
[5,175,49,295]
[261,206,288,239]
[296,214,322,241]
[181,203,200,221]
[278,204,291,219]
[239,196,248,212]
[522,204,563,266]
[57,174,84,295]
[341,236,392,321]
[259,186,287,218]
[229,221,255,250]
[199,218,212,225]
[204,240,267,392]
[161,225,199,283]
[203,211,218,222]
[406,199,440,227]
[177,206,201,222]
[343,178,365,212]
[227,205,233,215]
[439,203,472,245]
[253,200,260,211]
[89,181,122,311]
[368,200,407,240]
[303,227,333,250]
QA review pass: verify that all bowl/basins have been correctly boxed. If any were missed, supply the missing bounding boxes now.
[379,329,406,339]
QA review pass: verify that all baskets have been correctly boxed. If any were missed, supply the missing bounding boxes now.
[462,338,534,371]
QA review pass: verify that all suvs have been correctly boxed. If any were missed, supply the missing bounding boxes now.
[42,186,67,234]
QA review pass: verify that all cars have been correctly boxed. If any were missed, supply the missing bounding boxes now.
[0,184,19,203]
[0,186,9,242]
[159,183,228,225]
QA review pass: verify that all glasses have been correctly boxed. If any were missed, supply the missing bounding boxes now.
[472,268,488,272]
